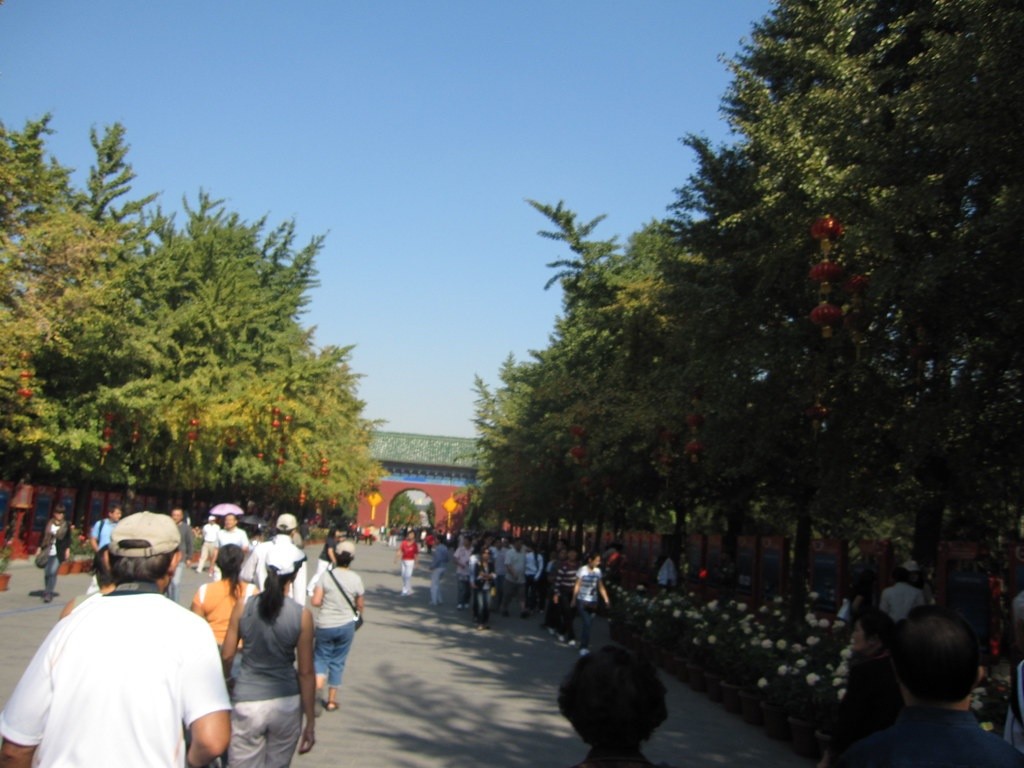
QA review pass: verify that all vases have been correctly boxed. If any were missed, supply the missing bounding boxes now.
[0,574,12,591]
[70,561,83,574]
[81,560,94,572]
[193,553,201,563]
[57,562,72,575]
[609,624,834,758]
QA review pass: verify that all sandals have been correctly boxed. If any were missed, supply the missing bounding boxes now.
[326,701,339,711]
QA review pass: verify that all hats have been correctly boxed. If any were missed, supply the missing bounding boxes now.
[265,544,307,575]
[335,541,356,558]
[901,561,921,571]
[108,510,181,557]
[208,515,216,522]
[275,513,298,531]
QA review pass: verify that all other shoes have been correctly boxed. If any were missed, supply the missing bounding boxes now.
[308,590,313,598]
[457,601,545,630]
[556,632,565,642]
[579,648,590,656]
[568,640,575,645]
[545,625,556,635]
[44,592,52,602]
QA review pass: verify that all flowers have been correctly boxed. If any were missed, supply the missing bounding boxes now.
[591,579,860,708]
[0,539,13,574]
[66,524,95,562]
[191,527,204,552]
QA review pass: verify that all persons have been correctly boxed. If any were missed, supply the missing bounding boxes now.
[827,553,1024,768]
[189,543,258,685]
[557,645,669,767]
[90,502,122,552]
[306,527,341,598]
[34,503,71,602]
[58,545,114,619]
[0,512,234,768]
[219,536,317,766]
[208,512,249,582]
[344,519,627,657]
[311,542,364,718]
[162,507,194,604]
[195,516,220,574]
[241,513,307,610]
[299,517,312,547]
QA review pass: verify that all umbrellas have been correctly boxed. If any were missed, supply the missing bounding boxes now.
[238,516,267,535]
[209,503,244,516]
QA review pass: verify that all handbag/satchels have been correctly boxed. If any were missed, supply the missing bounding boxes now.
[353,607,364,631]
[35,544,51,568]
